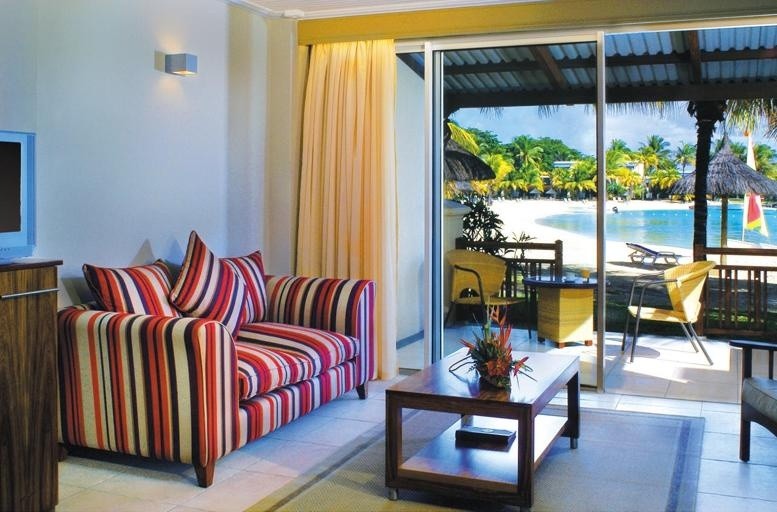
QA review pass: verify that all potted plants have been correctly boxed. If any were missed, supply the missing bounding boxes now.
[448,294,539,394]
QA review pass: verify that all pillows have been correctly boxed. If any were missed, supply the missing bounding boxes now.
[82,230,270,342]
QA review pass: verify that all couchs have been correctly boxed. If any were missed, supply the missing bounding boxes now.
[57,274,378,488]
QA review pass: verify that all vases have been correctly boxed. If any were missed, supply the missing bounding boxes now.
[480,371,509,388]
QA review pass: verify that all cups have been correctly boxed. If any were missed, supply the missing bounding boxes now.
[581,268,590,282]
[566,272,579,280]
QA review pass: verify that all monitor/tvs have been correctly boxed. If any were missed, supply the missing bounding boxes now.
[0,130,37,265]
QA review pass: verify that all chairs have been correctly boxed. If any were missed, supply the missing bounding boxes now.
[444,249,532,340]
[621,260,718,367]
[626,242,692,265]
[728,339,777,463]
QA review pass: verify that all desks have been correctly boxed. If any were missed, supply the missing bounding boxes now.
[522,275,612,349]
[1,257,64,512]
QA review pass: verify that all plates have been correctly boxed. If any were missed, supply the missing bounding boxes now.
[563,279,578,281]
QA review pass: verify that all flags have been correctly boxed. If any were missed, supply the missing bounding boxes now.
[743,132,768,237]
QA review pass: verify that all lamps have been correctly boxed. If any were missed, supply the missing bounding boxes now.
[164,53,198,76]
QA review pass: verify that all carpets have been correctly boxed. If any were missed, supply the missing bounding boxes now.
[241,403,707,512]
